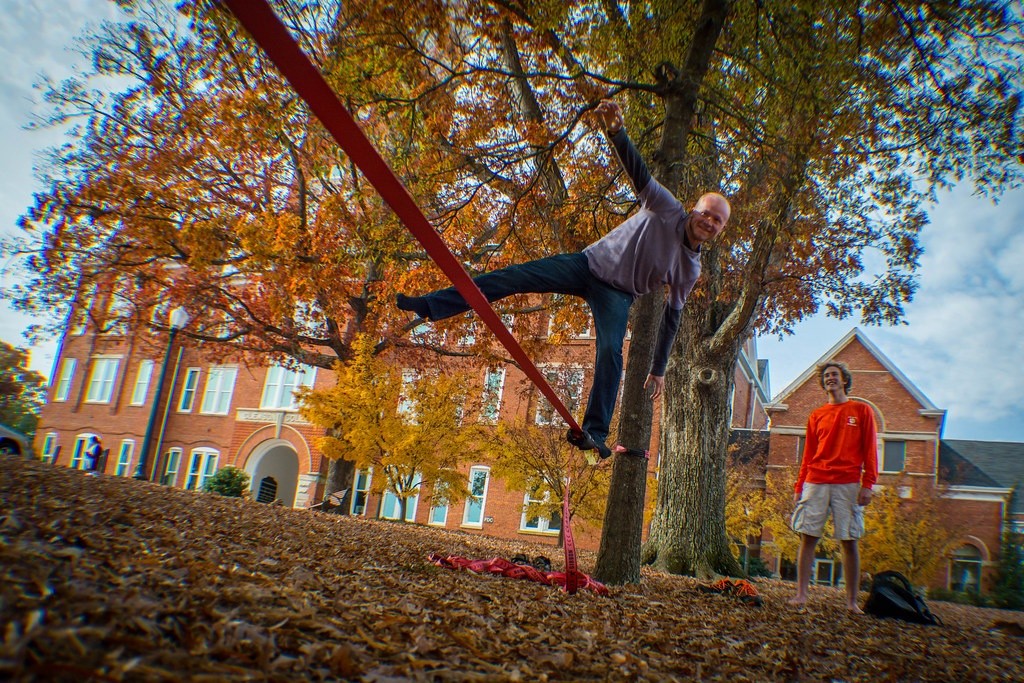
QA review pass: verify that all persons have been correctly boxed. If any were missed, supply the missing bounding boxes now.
[394,96,732,459]
[84,435,104,472]
[788,359,881,615]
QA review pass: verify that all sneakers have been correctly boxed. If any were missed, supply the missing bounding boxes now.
[695,575,737,596]
[733,579,764,607]
[510,552,552,573]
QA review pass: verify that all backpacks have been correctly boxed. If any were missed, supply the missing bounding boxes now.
[862,570,944,627]
